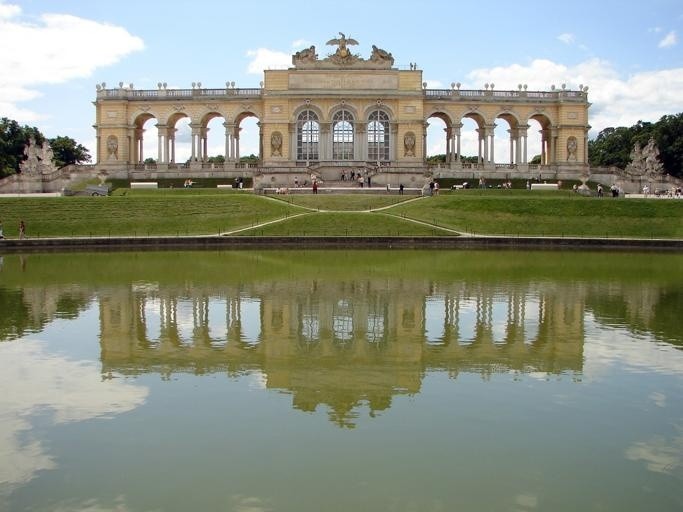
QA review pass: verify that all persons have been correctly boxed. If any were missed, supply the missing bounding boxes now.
[463,181,468,189]
[295,176,299,188]
[19,220,25,240]
[234,176,243,189]
[643,184,648,198]
[558,179,562,190]
[597,184,603,198]
[0,220,4,238]
[502,180,512,190]
[184,178,193,188]
[429,182,434,195]
[311,173,317,185]
[434,181,439,195]
[509,162,514,169]
[666,186,682,199]
[611,184,618,197]
[313,181,320,194]
[400,184,404,195]
[573,184,578,191]
[340,161,384,188]
[276,186,290,195]
[479,176,487,189]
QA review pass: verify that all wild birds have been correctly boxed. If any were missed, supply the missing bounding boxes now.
[326,32,359,50]
